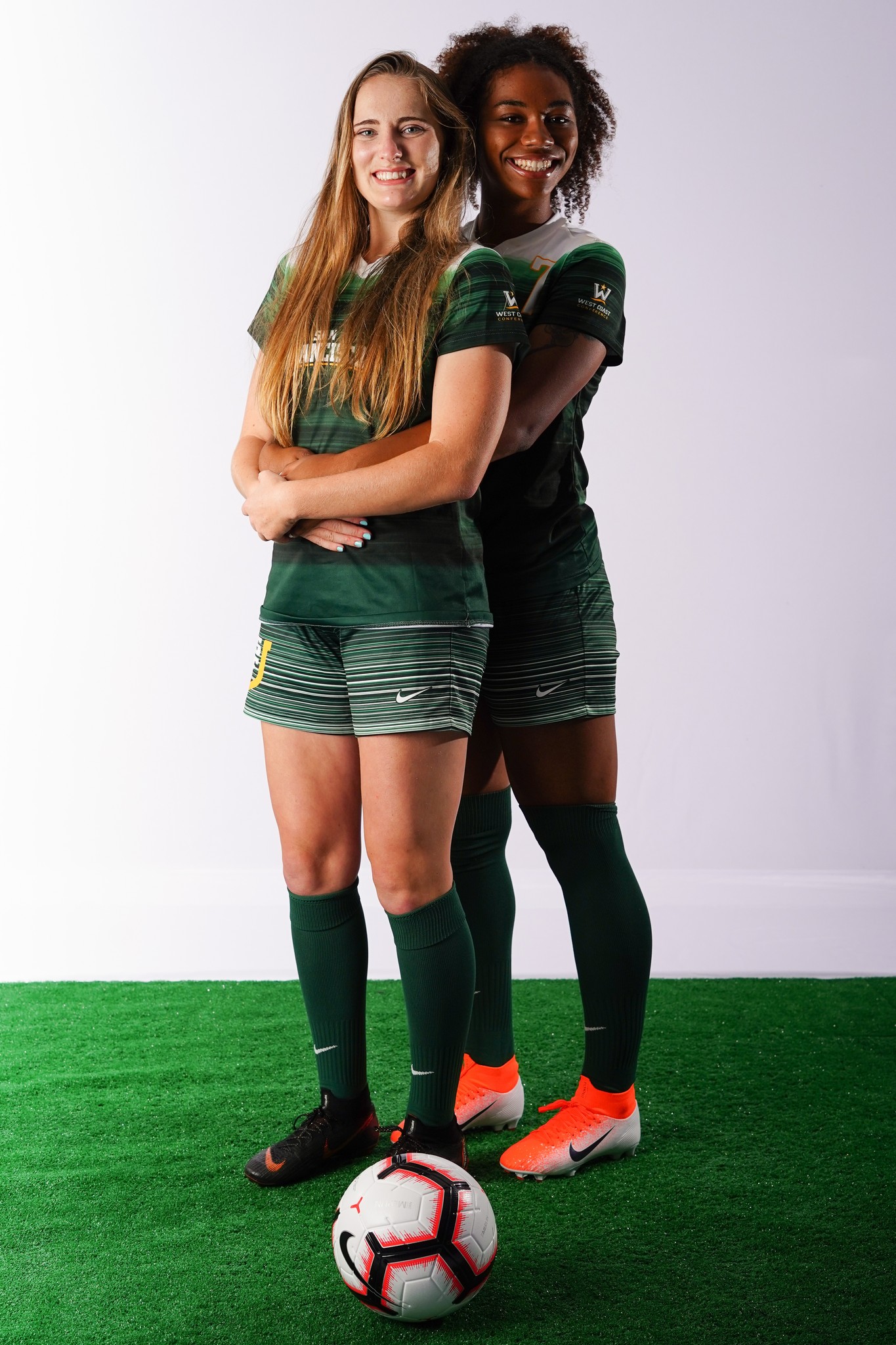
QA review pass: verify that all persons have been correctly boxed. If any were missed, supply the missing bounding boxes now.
[230,50,531,1188]
[259,24,641,1181]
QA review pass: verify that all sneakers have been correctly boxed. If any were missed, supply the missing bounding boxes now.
[390,1053,525,1144]
[381,1114,469,1171]
[244,1087,380,1187]
[499,1074,641,1180]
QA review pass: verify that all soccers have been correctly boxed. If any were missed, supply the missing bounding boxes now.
[330,1149,500,1328]
